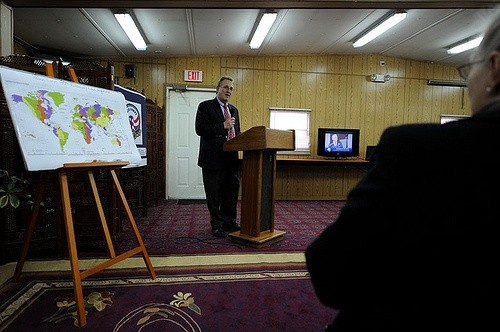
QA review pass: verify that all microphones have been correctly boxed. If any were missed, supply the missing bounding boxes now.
[230,107,236,131]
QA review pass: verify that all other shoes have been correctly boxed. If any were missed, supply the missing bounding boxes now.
[222,223,241,231]
[212,227,227,236]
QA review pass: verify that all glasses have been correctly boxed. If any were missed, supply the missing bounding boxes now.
[457,60,483,79]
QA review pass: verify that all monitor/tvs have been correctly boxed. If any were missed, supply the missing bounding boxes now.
[317,128,360,160]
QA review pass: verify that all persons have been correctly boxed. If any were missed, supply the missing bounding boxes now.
[329,133,343,150]
[305,14,499,332]
[195,76,243,238]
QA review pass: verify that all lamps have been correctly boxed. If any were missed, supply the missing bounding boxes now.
[249,11,277,50]
[352,10,408,48]
[114,9,148,51]
[446,32,483,55]
[370,73,390,83]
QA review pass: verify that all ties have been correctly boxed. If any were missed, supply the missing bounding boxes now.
[223,103,234,140]
